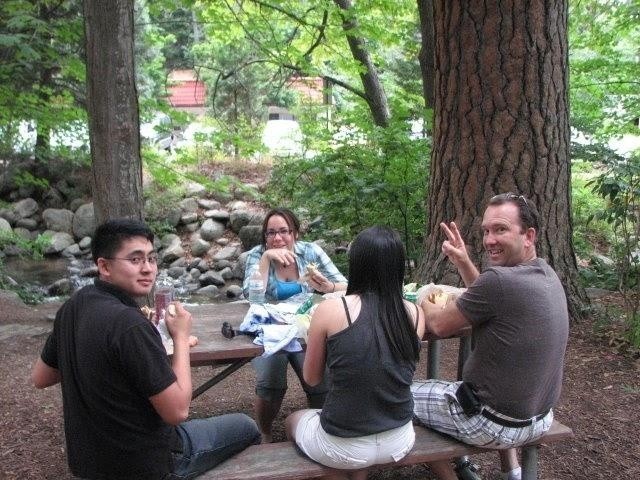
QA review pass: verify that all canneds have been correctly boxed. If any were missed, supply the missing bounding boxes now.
[403,292,419,304]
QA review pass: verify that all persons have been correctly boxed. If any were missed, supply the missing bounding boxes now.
[410,191,570,480]
[241,206,349,443]
[30,216,261,480]
[285,223,425,480]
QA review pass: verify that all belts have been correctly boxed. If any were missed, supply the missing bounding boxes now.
[482,408,550,428]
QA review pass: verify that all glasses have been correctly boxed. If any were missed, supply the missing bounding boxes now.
[108,256,157,263]
[266,229,292,238]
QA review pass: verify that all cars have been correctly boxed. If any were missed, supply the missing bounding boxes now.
[139,111,175,153]
[262,119,307,157]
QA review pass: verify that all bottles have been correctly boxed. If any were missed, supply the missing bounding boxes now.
[153,268,176,325]
[247,264,265,304]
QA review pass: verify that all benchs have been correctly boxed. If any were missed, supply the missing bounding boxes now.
[184,420,576,480]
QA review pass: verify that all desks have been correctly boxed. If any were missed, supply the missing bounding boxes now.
[142,291,481,407]
